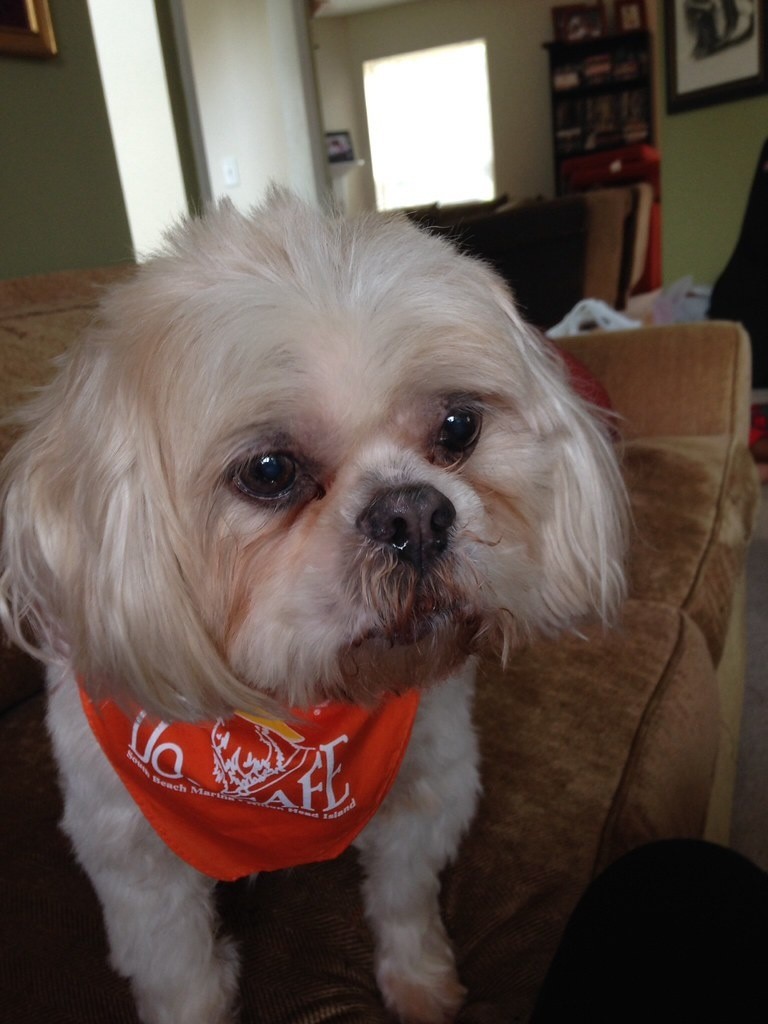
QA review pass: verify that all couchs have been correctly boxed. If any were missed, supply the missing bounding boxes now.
[1,264,760,1024]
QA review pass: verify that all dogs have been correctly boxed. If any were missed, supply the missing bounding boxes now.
[0,174,658,1024]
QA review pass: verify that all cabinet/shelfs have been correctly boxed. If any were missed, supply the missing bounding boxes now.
[542,4,667,293]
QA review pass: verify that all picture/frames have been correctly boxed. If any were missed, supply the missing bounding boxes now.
[663,1,768,116]
